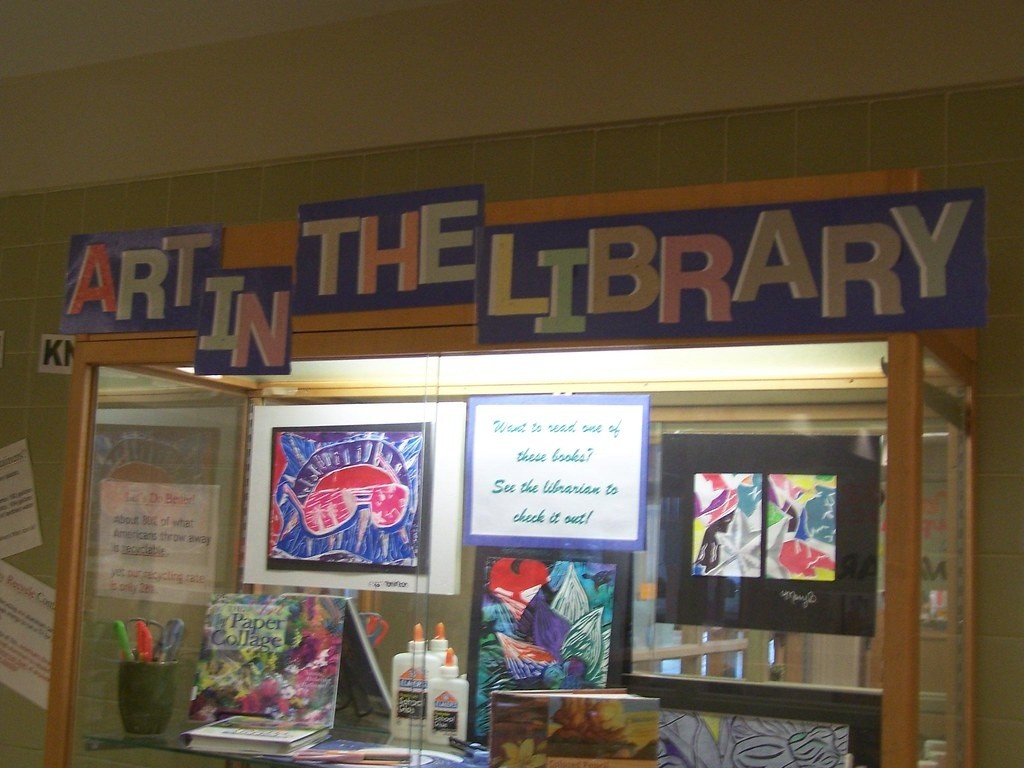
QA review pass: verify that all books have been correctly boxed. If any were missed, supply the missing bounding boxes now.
[339,594,392,716]
[489,689,660,768]
[186,591,346,731]
[178,716,333,762]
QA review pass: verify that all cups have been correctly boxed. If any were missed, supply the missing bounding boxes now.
[117,660,179,735]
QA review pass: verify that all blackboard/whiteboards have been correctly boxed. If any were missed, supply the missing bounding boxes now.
[462,393,651,552]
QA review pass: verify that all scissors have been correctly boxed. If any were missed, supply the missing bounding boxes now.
[127,618,164,656]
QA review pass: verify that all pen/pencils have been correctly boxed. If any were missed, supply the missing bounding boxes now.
[110,614,186,665]
